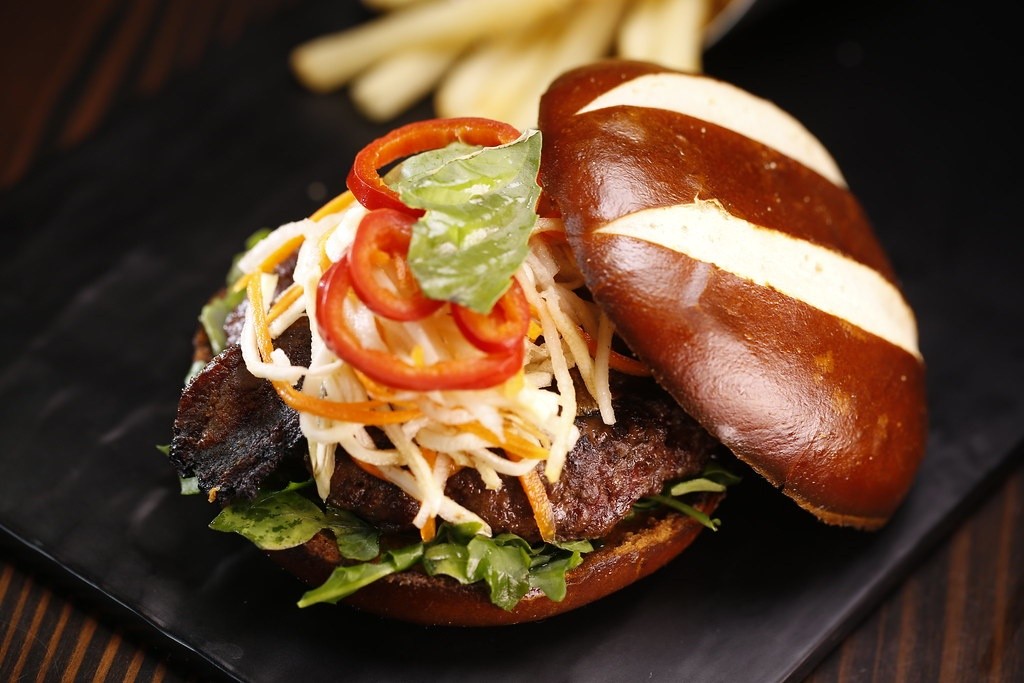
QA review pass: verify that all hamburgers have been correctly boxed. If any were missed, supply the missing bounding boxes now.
[162,61,928,627]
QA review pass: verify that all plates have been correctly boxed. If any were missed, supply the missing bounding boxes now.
[0,1,1024,683]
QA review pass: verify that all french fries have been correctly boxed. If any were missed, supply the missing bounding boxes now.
[288,0,715,129]
